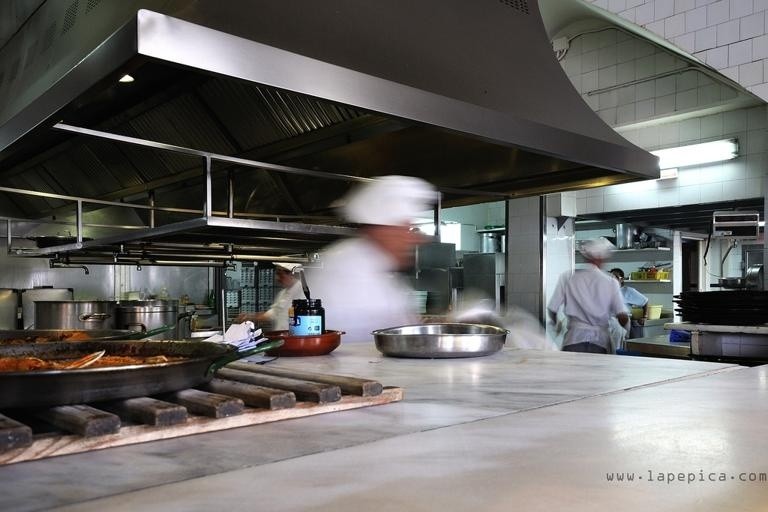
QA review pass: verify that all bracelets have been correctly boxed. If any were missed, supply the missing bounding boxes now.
[643,316,648,318]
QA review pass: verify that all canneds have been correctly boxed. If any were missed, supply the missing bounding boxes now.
[289,299,325,337]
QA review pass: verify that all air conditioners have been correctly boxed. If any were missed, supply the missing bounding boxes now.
[648,136,742,170]
[712,209,760,242]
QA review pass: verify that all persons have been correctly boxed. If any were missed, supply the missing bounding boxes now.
[236,255,309,332]
[609,267,650,340]
[546,236,632,354]
[288,175,438,344]
[454,310,557,350]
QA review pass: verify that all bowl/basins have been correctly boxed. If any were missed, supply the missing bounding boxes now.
[718,277,746,288]
[647,305,662,320]
[371,322,510,359]
[257,331,346,356]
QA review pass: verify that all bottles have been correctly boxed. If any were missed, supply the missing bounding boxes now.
[208,289,216,306]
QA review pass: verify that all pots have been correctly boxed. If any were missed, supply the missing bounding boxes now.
[0,300,285,406]
[612,223,641,249]
[673,290,768,325]
[479,233,499,254]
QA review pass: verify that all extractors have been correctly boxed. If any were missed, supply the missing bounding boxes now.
[2,2,660,264]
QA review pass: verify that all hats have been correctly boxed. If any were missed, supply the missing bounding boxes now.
[272,262,302,273]
[578,237,614,261]
[345,176,441,227]
